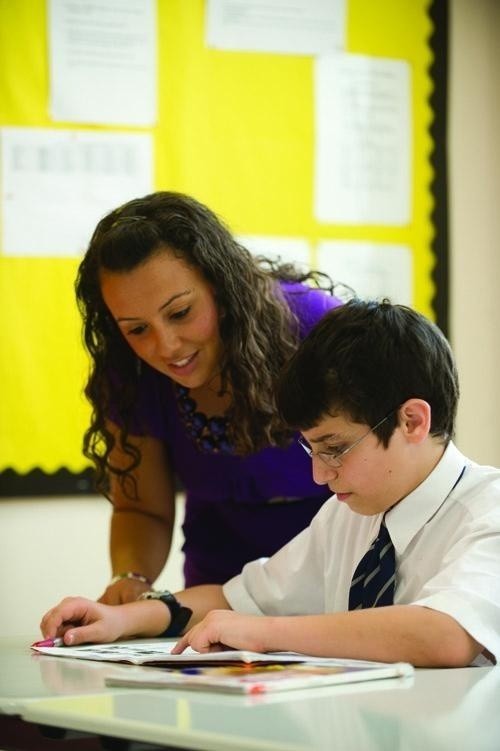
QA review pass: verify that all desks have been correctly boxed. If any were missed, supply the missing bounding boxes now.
[1,624,500,751]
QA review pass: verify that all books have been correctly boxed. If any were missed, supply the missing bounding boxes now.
[31,621,346,664]
[105,655,415,694]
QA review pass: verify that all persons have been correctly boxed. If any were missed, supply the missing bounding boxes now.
[72,189,363,610]
[40,297,499,669]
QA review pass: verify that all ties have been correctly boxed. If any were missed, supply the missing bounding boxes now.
[346,529,393,613]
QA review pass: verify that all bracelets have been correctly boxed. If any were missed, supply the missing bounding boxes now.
[105,571,153,588]
[135,585,194,638]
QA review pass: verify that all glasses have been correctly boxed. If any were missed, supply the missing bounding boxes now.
[286,404,394,466]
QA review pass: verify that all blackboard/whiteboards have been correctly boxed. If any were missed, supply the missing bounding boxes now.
[1,3,443,494]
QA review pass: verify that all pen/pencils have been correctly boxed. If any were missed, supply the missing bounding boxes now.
[32,638,64,648]
